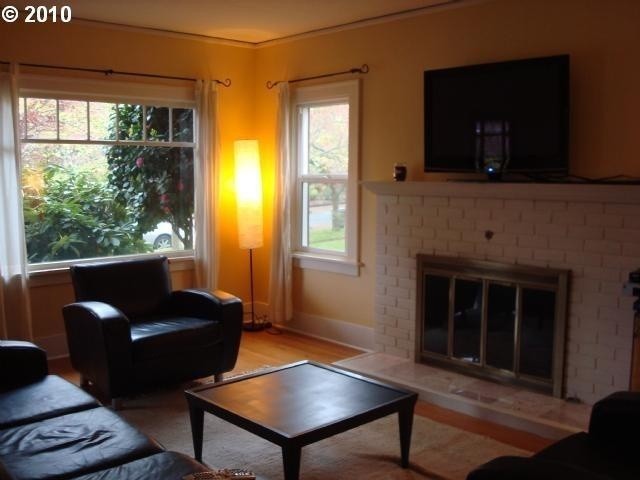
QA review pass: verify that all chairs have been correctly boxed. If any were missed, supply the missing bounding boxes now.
[466,391,640,480]
[61,254,243,410]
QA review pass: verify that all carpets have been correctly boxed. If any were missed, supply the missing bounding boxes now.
[115,363,536,480]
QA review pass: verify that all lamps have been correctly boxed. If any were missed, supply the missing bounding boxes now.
[233,139,272,332]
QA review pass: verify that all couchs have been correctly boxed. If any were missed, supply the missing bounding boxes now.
[1,341,258,480]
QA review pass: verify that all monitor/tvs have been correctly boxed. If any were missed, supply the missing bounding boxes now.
[422,53,573,181]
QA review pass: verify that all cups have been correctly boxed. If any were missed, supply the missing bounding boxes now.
[393,160,408,182]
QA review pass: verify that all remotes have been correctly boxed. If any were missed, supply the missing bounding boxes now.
[180,469,257,479]
